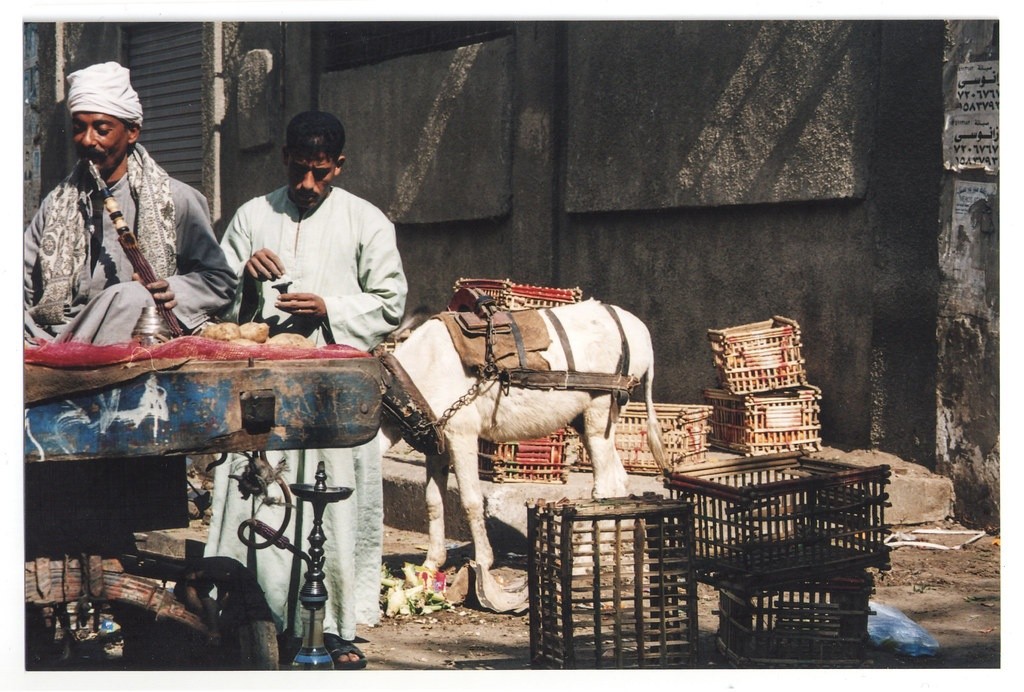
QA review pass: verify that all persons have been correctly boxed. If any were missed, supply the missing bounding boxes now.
[24,62,239,337]
[203,110,408,670]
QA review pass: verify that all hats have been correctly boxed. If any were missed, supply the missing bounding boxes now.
[64,61,144,131]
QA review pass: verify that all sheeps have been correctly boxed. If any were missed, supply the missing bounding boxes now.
[377,300,670,573]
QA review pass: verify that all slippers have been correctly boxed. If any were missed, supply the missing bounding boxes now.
[324,634,366,670]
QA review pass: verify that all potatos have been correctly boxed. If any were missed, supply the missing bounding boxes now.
[197,321,316,349]
